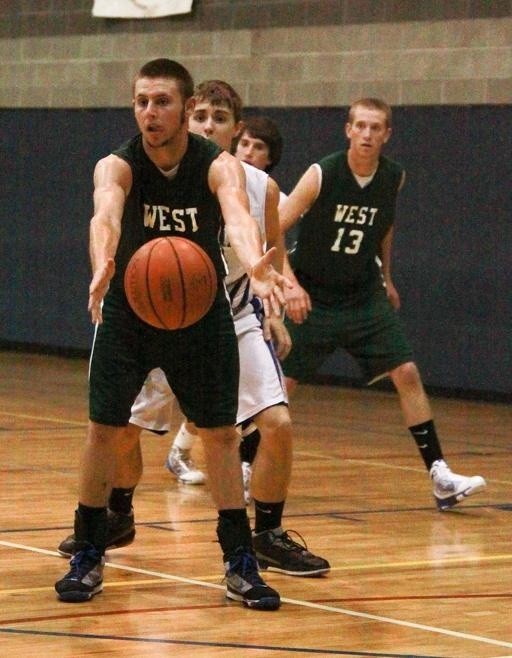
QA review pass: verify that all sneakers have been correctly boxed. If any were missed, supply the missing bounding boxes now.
[56,506,137,558]
[251,531,332,578]
[429,460,485,511]
[222,549,280,610]
[164,444,207,485]
[240,462,256,506]
[55,542,106,602]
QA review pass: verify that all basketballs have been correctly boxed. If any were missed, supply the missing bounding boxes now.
[124,236,218,331]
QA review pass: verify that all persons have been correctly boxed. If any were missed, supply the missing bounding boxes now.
[56,59,331,609]
[239,98,487,513]
